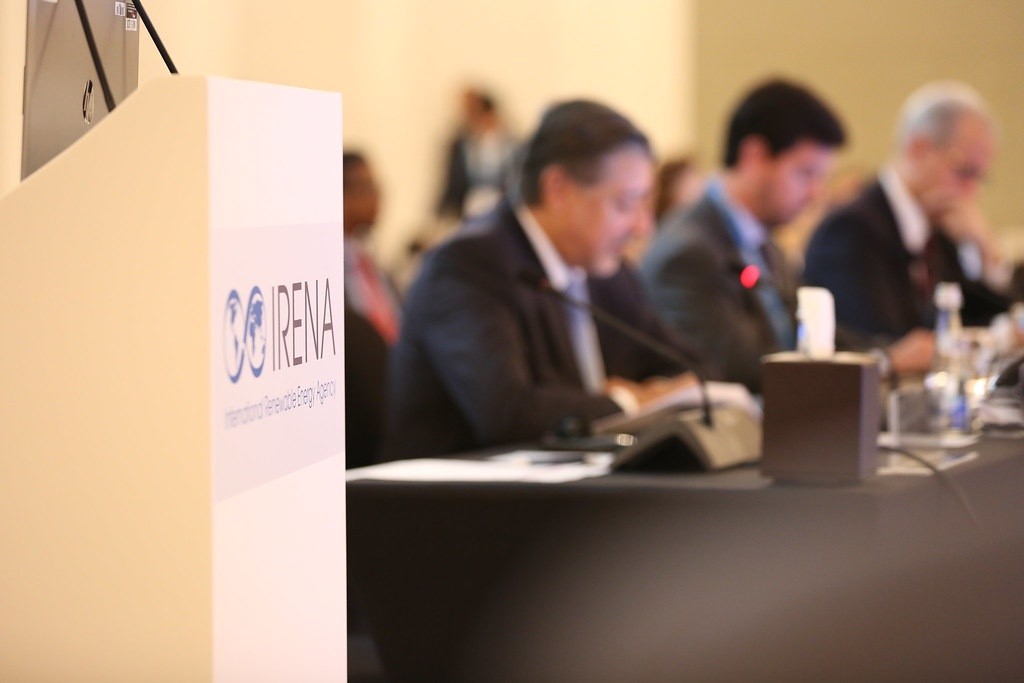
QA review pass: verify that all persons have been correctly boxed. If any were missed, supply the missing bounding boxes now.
[379,98,699,460]
[805,85,1024,387]
[436,93,518,230]
[343,151,401,470]
[639,80,936,394]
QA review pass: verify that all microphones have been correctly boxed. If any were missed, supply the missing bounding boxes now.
[509,267,766,469]
[724,259,797,354]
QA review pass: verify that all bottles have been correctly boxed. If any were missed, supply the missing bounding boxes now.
[924,282,985,445]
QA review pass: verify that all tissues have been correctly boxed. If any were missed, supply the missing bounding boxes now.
[754,287,883,485]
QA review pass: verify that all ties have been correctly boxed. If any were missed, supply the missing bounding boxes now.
[926,234,943,291]
[760,239,799,310]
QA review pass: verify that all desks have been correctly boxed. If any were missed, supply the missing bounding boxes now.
[346,367,1024,683]
